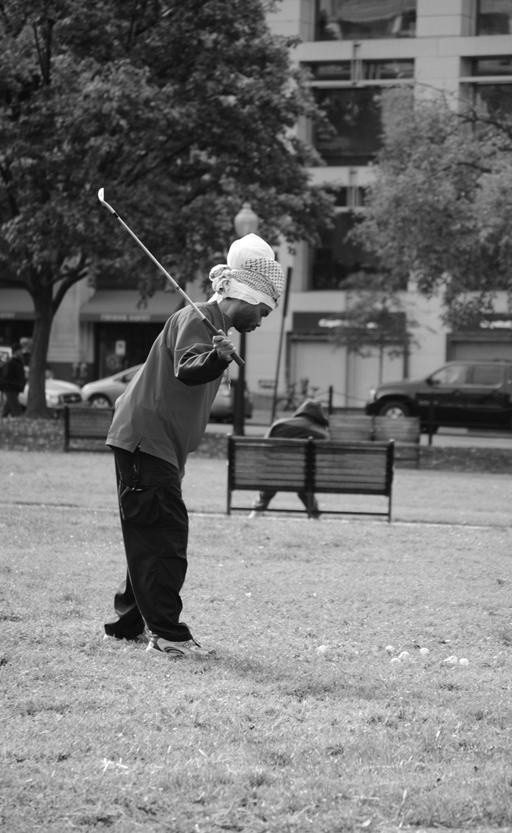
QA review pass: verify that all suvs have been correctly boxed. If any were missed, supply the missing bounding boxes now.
[366,358,511,436]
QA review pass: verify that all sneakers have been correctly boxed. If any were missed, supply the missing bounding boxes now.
[103,628,216,660]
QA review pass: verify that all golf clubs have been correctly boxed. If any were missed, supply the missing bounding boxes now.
[97,187,246,369]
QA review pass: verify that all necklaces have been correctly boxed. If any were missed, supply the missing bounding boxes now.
[215,300,232,389]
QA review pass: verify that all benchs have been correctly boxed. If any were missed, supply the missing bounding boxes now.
[226,433,395,522]
[326,413,421,464]
[63,404,118,451]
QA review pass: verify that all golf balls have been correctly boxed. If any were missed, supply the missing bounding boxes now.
[449,655,458,665]
[317,645,327,655]
[385,645,409,666]
[419,648,430,656]
[459,658,469,667]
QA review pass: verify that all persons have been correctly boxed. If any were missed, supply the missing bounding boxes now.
[253,396,331,517]
[1,341,28,419]
[99,229,289,661]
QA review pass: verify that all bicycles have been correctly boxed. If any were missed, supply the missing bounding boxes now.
[272,381,331,421]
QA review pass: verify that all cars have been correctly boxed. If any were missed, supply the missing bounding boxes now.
[1,347,86,413]
[77,359,257,425]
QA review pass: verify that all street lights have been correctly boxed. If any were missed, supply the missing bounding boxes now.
[231,202,260,436]
